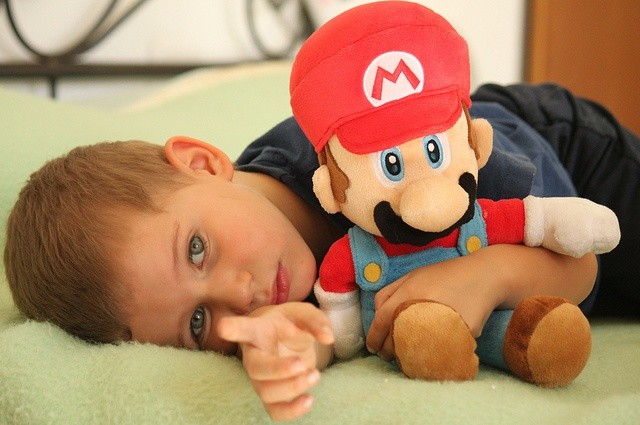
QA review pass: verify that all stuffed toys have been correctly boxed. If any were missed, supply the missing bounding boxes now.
[290,1,621,390]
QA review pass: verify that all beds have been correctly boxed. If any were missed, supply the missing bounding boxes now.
[1,1,639,424]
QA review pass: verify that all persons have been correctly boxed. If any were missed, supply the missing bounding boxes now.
[2,81,639,423]
